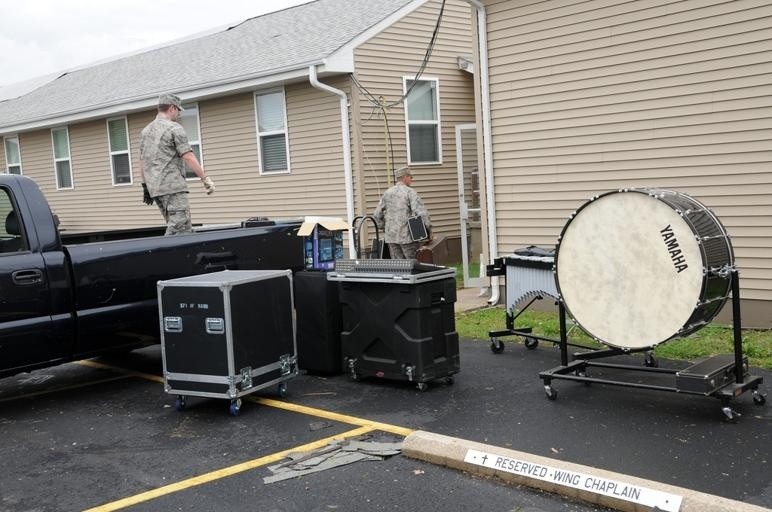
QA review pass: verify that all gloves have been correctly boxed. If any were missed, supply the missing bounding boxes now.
[202,175,216,196]
[142,182,153,206]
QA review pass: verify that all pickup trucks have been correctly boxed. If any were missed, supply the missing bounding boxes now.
[1,166,310,393]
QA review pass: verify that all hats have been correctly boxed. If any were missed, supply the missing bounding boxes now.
[158,91,186,113]
[393,166,414,178]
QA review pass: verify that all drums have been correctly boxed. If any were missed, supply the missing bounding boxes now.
[554,186,732,352]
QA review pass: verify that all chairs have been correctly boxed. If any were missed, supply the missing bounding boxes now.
[1,209,22,253]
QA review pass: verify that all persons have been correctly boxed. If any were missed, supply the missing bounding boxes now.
[373,164,434,261]
[139,90,217,238]
